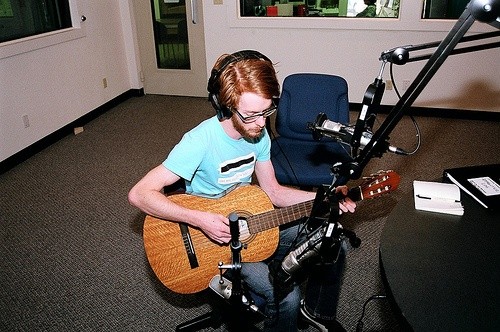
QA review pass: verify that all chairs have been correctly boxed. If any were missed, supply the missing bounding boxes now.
[266,73,353,191]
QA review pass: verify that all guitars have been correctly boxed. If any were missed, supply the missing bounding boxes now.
[142,169,400,295]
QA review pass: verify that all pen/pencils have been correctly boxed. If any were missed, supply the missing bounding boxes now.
[416,194,462,202]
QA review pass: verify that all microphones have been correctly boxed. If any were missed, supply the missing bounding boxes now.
[311,112,406,155]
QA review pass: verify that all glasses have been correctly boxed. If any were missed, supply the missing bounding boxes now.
[231,100,277,123]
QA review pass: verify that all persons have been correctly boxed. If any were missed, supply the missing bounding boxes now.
[127,50,357,332]
[356,0,376,17]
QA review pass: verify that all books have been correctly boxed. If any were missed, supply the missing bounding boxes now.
[444,164,500,209]
[412,180,464,216]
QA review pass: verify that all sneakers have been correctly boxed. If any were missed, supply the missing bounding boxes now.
[300,299,346,332]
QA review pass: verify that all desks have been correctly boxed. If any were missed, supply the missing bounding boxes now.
[357,176,500,332]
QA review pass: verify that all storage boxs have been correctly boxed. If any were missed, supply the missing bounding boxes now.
[266,4,293,17]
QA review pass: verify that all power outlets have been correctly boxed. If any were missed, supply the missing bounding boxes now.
[386,80,393,90]
[103,78,107,89]
[22,114,29,127]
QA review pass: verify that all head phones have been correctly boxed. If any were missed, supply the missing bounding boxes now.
[207,49,272,119]
